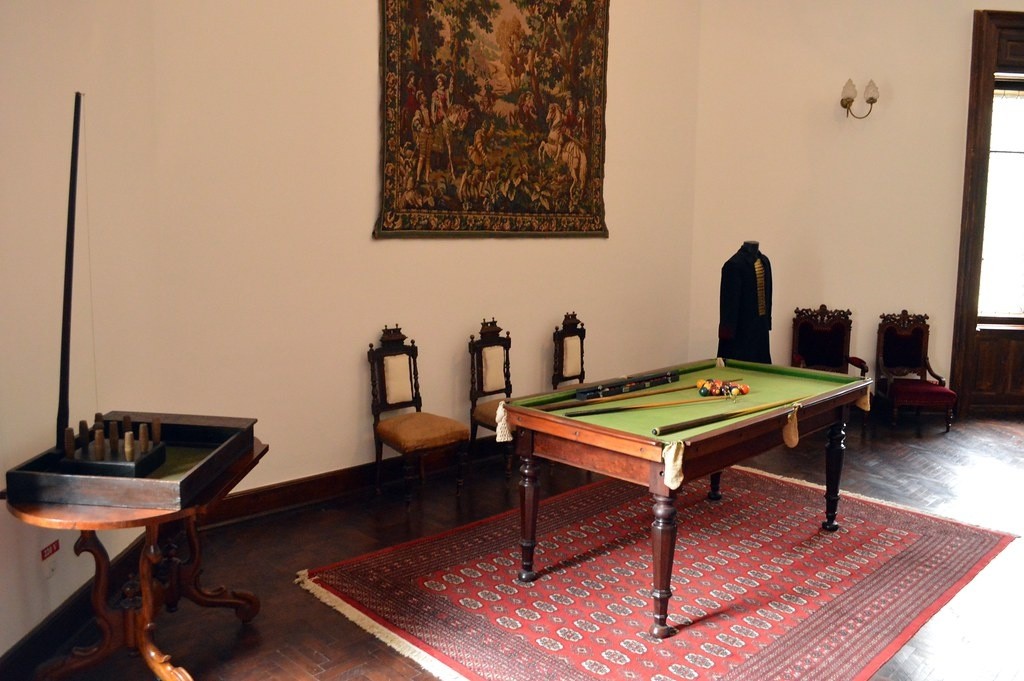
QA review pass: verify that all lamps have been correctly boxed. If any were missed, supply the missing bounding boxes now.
[840,79,880,120]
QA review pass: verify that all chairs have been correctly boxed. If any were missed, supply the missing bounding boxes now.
[791,305,874,442]
[874,310,957,432]
[368,324,472,514]
[467,317,557,502]
[552,310,594,481]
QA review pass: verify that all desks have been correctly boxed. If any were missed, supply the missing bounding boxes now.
[501,356,876,640]
[1,436,271,681]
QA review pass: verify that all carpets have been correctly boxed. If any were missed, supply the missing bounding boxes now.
[294,464,1024,681]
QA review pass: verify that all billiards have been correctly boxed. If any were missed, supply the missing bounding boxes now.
[696,378,750,397]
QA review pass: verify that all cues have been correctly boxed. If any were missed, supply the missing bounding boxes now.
[564,395,733,417]
[651,395,812,437]
[529,377,744,411]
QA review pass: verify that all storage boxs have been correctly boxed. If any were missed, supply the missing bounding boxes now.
[7,409,259,512]
